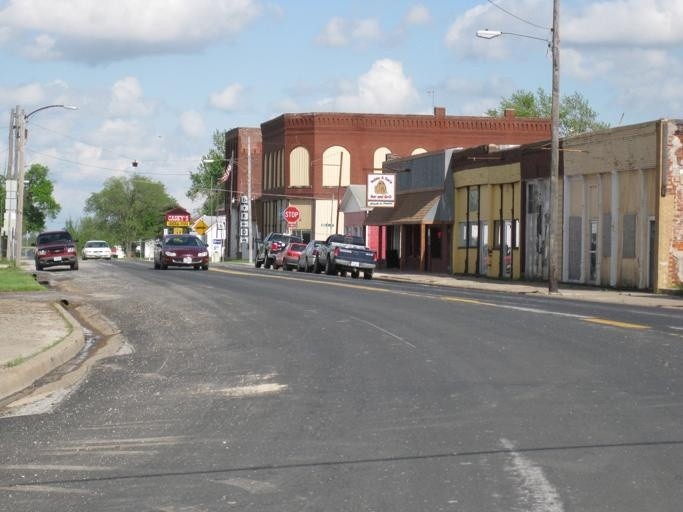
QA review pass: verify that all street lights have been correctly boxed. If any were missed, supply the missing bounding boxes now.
[204,159,253,264]
[476,30,560,293]
[15,105,77,267]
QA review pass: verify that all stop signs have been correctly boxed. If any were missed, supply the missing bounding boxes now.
[283,207,300,224]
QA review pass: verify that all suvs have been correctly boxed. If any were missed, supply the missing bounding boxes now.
[31,231,79,270]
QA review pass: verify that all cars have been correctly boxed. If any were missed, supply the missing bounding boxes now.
[82,240,112,260]
[154,234,209,270]
[255,232,377,279]
